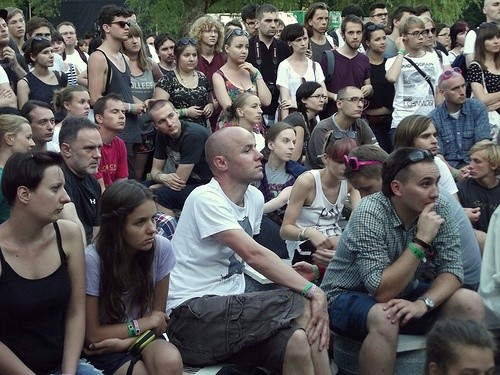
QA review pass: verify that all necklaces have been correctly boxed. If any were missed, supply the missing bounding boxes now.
[178,71,195,88]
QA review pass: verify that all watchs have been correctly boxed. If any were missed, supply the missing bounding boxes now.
[418,295,435,313]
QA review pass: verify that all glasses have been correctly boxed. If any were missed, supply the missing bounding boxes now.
[30,36,50,54]
[390,149,434,182]
[425,27,436,35]
[344,155,382,170]
[406,30,428,38]
[324,130,358,153]
[174,38,198,51]
[439,67,462,85]
[340,97,367,106]
[437,33,451,37]
[243,22,257,29]
[371,13,388,18]
[225,29,249,41]
[310,95,327,99]
[111,20,131,29]
[365,23,385,31]
[478,21,498,30]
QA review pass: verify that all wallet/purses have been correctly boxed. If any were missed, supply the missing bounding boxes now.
[128,328,156,357]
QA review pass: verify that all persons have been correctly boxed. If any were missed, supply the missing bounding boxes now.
[0,0,500,375]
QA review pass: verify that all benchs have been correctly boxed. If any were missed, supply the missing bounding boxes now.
[331,329,429,375]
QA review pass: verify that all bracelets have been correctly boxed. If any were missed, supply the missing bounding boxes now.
[307,284,317,299]
[302,282,314,296]
[153,171,165,182]
[178,108,185,116]
[453,169,459,178]
[185,108,187,116]
[126,320,137,337]
[408,243,427,263]
[255,75,263,79]
[299,227,306,241]
[397,49,407,55]
[253,70,259,79]
[412,238,434,253]
[133,320,140,335]
[130,104,135,113]
[313,265,319,280]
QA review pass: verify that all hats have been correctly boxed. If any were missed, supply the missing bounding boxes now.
[0,9,9,22]
